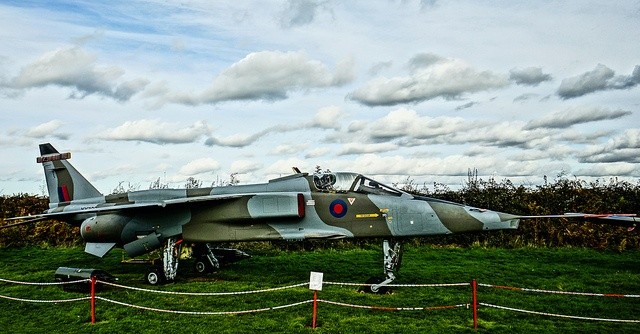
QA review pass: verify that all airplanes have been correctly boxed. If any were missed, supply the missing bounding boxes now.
[0,142,640,294]
